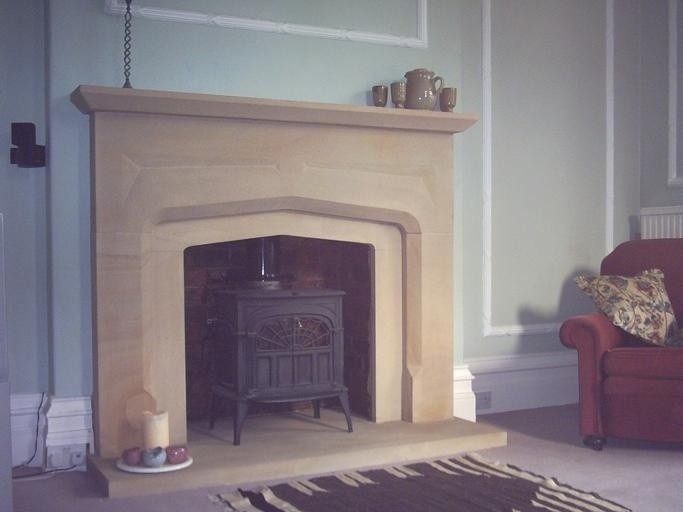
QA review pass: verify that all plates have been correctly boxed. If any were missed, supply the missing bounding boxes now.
[116,455,193,474]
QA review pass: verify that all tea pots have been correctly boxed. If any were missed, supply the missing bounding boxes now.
[404,68,444,110]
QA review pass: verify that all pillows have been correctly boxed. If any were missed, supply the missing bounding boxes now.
[574,268,682,348]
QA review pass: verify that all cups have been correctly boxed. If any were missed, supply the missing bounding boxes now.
[141,411,168,447]
[440,87,456,111]
[372,85,387,107]
[390,82,406,108]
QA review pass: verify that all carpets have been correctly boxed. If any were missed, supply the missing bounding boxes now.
[206,451,634,512]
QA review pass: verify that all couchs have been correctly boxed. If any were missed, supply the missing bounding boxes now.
[559,238,683,450]
